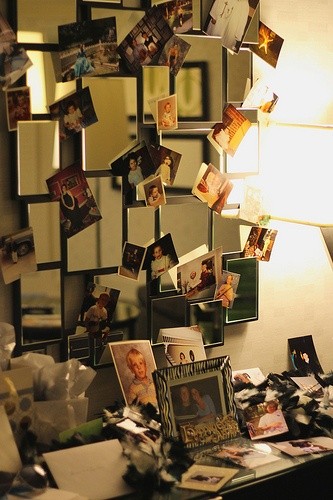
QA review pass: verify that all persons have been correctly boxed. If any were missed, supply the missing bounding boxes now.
[151,242,176,279]
[0,0,328,487]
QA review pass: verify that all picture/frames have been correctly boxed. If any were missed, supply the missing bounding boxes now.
[152,355,242,451]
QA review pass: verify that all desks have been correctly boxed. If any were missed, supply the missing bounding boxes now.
[0,410,333,500]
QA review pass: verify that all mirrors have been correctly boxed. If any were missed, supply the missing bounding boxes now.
[16,0,259,371]
